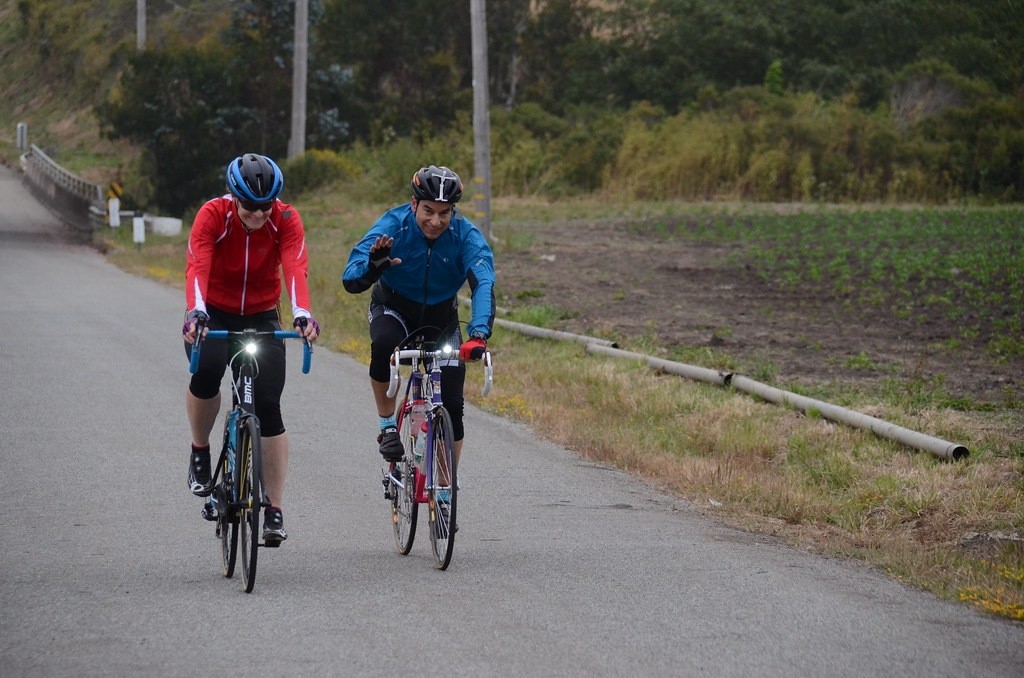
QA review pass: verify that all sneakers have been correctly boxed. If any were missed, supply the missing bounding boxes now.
[262,506,287,541]
[188,452,212,497]
[435,501,458,539]
[376,425,404,457]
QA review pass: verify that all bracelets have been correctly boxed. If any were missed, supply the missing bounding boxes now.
[470,332,487,340]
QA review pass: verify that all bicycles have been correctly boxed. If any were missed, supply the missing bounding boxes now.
[383,320,494,572]
[190,312,314,593]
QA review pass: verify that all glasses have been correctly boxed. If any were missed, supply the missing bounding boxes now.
[237,196,273,211]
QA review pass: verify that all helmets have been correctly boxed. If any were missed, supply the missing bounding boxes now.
[226,153,284,201]
[412,165,463,202]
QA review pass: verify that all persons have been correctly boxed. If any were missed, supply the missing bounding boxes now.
[183,153,320,541]
[342,167,497,534]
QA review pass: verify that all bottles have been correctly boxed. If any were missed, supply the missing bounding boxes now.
[411,399,428,469]
[230,405,238,471]
